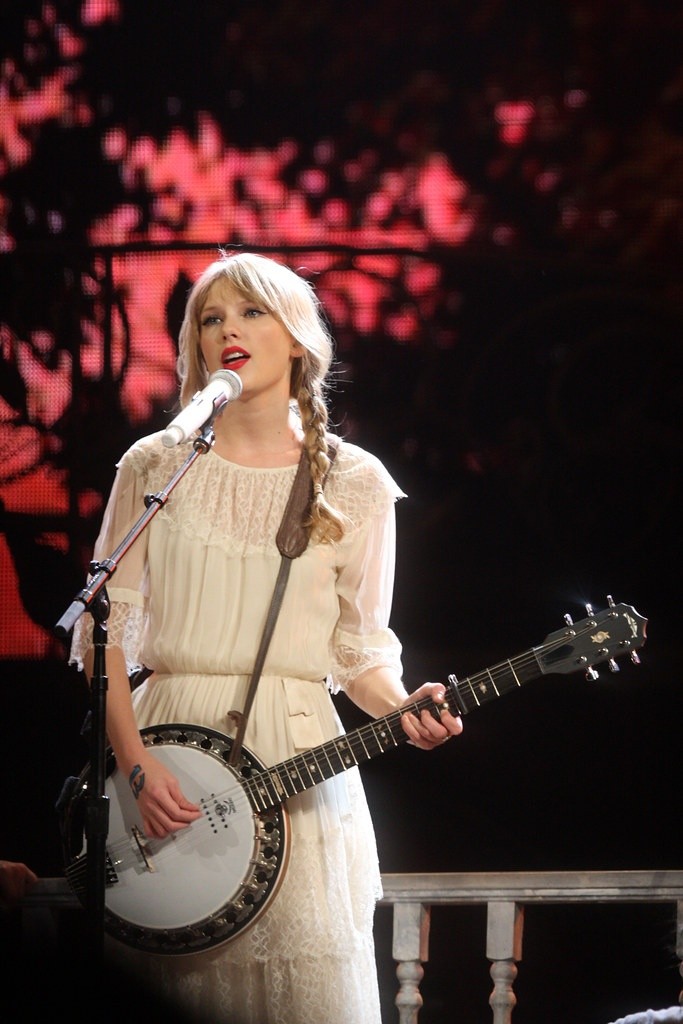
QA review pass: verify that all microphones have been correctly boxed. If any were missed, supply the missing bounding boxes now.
[162,370,242,449]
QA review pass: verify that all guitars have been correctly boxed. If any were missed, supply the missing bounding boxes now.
[104,588,647,956]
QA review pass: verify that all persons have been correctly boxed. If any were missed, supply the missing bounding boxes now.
[68,253,463,1024]
